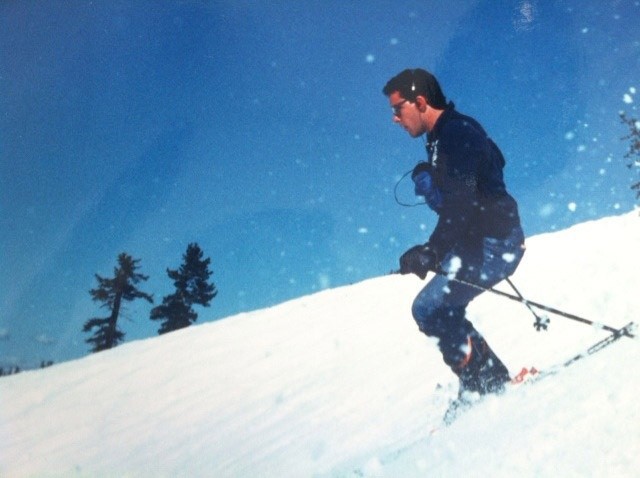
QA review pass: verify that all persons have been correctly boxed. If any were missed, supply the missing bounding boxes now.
[381,66,527,407]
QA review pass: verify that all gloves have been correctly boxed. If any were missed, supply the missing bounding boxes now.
[399,241,442,279]
[412,162,443,212]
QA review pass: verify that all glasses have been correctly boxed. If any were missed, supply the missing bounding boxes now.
[391,99,409,116]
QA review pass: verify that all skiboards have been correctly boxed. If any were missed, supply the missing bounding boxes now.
[444,321,633,420]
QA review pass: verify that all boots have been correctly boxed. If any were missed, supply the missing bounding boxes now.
[472,328,513,382]
[443,333,507,424]
